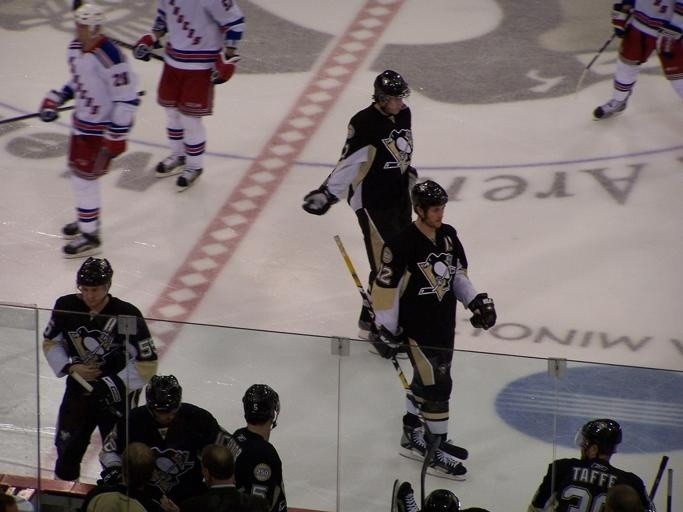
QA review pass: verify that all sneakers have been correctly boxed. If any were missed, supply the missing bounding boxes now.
[400,424,466,475]
[593,98,626,117]
[156,154,202,186]
[63,222,99,253]
[396,482,419,512]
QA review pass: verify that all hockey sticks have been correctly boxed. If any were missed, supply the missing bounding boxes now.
[334,235,468,459]
[421,438,441,512]
[575,10,634,94]
[72,1,165,61]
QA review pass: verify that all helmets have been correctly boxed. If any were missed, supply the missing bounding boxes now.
[426,490,460,512]
[374,70,410,99]
[77,257,113,286]
[145,375,181,411]
[574,419,622,454]
[412,181,447,211]
[74,3,107,26]
[242,384,280,428]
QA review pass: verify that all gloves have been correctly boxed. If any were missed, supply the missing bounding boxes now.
[303,188,335,215]
[610,3,629,38]
[210,52,240,84]
[40,89,62,122]
[657,32,673,59]
[368,327,403,359]
[132,33,157,61]
[468,293,496,330]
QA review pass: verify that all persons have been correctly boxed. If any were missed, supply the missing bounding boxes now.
[391,479,488,511]
[0,493,18,511]
[136,0,247,191]
[214,382,290,511]
[528,418,657,512]
[369,180,502,481]
[606,485,644,511]
[98,372,228,493]
[39,257,158,483]
[591,0,683,122]
[298,69,414,346]
[81,443,271,511]
[34,0,134,259]
